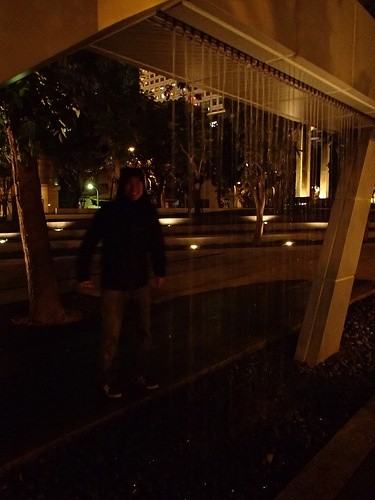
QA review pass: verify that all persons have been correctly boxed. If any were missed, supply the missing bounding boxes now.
[77,167,168,397]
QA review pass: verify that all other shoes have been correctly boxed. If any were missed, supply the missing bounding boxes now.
[128,367,160,390]
[93,382,123,398]
